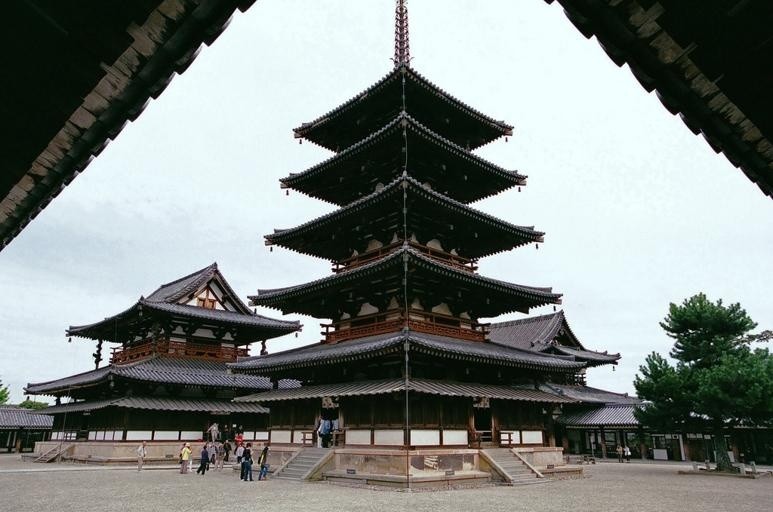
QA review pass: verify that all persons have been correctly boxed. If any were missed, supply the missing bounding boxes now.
[137,441,147,472]
[316,414,339,448]
[180,423,269,481]
[623,444,630,463]
[616,444,623,463]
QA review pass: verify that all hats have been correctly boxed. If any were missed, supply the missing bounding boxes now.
[184,443,191,447]
